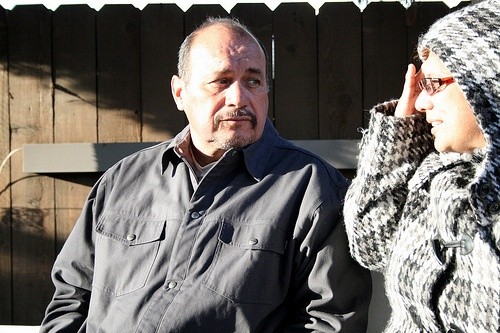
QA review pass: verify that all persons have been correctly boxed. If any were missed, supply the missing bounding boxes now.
[43,18,363,333]
[341,0,500,333]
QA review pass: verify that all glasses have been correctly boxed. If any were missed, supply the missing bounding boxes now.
[416,76,454,94]
